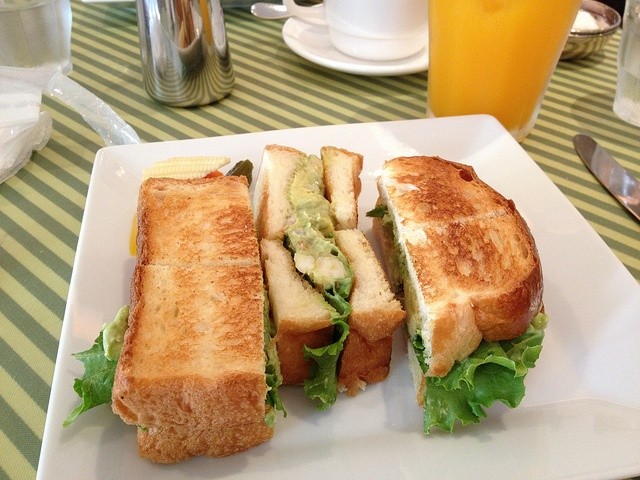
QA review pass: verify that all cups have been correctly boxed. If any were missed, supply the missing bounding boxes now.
[1,1,73,75]
[281,1,426,59]
[613,0,639,128]
[423,0,580,145]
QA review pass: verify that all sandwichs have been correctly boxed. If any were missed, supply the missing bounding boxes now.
[62,175,286,464]
[366,155,550,434]
[254,144,406,412]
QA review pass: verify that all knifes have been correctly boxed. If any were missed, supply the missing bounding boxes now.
[574,135,640,222]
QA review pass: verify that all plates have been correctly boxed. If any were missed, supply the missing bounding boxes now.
[283,3,428,76]
[36,114,640,479]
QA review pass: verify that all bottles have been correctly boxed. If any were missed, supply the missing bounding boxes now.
[135,0,235,106]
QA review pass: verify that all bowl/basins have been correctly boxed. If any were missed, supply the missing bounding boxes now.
[562,2,622,59]
